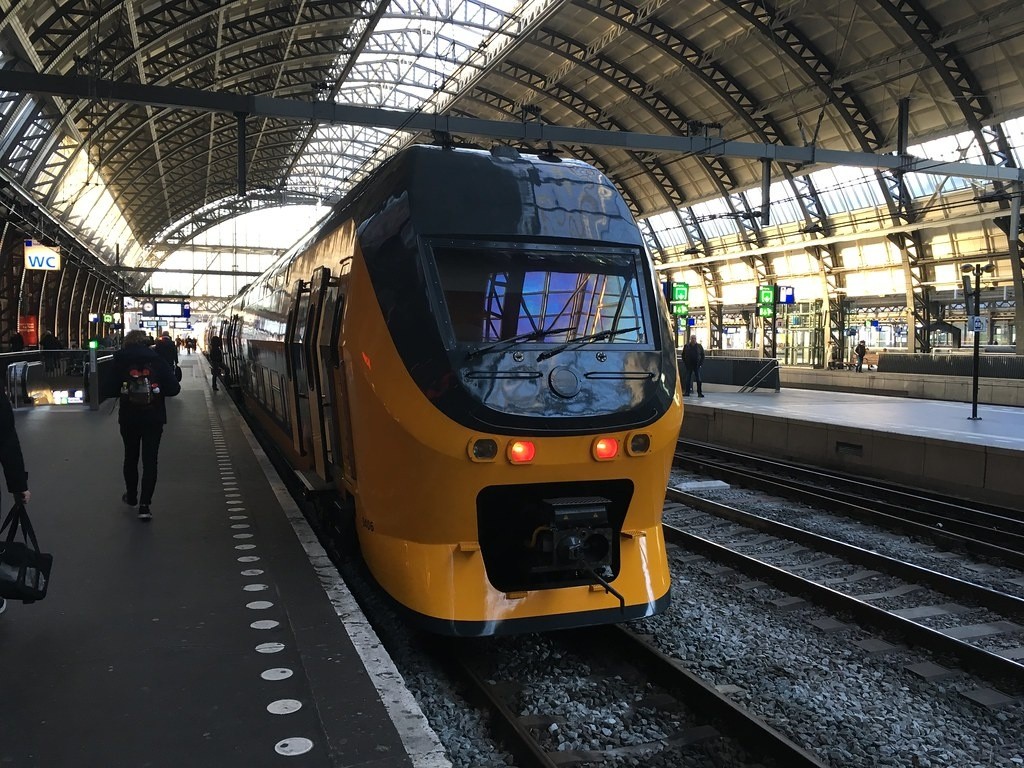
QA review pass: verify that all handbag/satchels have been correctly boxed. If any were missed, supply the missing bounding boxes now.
[0,493,53,603]
[175,365,182,382]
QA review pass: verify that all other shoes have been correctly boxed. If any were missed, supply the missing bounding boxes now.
[212,384,219,390]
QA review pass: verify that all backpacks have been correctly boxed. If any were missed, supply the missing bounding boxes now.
[121,358,157,405]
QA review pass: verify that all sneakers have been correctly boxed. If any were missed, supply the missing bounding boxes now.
[0,599,6,614]
[138,505,153,519]
[122,494,138,508]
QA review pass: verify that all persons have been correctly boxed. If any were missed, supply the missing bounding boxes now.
[8,327,196,384]
[0,380,30,614]
[102,330,180,519]
[681,335,705,397]
[209,336,223,390]
[853,340,866,372]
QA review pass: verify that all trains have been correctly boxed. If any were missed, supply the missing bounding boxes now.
[206,146,687,644]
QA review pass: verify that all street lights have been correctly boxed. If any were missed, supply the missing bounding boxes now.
[960,263,995,421]
